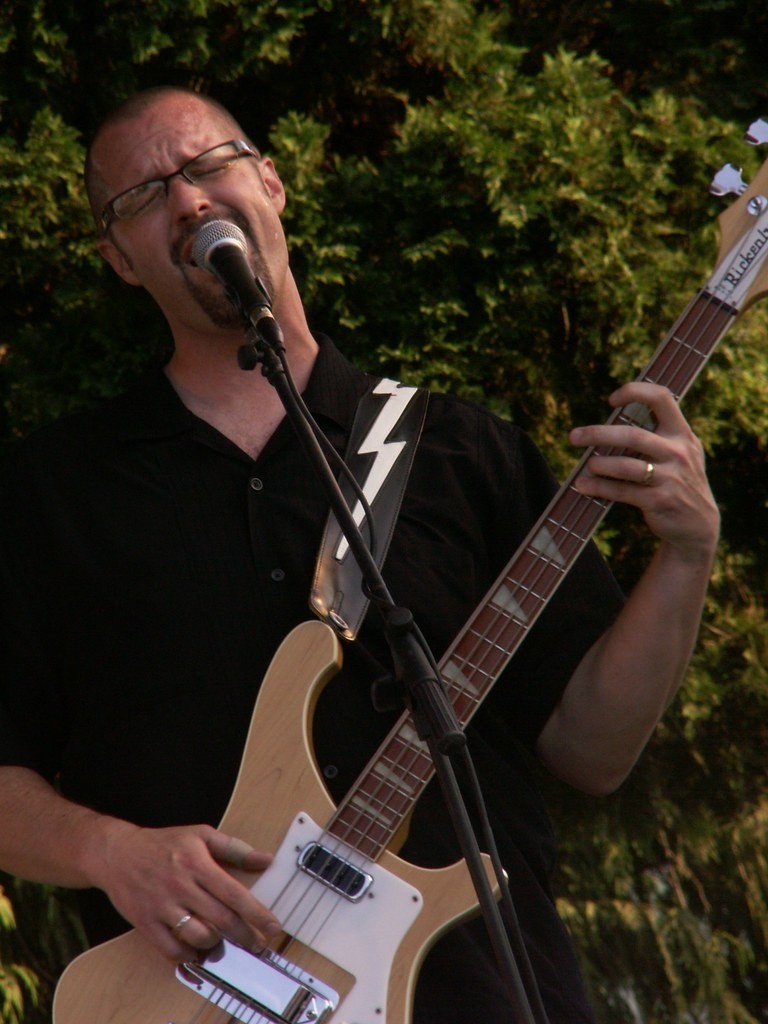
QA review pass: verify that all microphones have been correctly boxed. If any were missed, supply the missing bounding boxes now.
[192,220,287,356]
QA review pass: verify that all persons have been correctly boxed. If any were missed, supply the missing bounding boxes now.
[0,84,719,1024]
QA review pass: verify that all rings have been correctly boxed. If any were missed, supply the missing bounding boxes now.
[172,913,192,936]
[644,460,655,485]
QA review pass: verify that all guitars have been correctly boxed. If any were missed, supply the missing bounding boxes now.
[44,114,768,1024]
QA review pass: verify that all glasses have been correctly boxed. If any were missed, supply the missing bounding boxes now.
[100,139,262,240]
